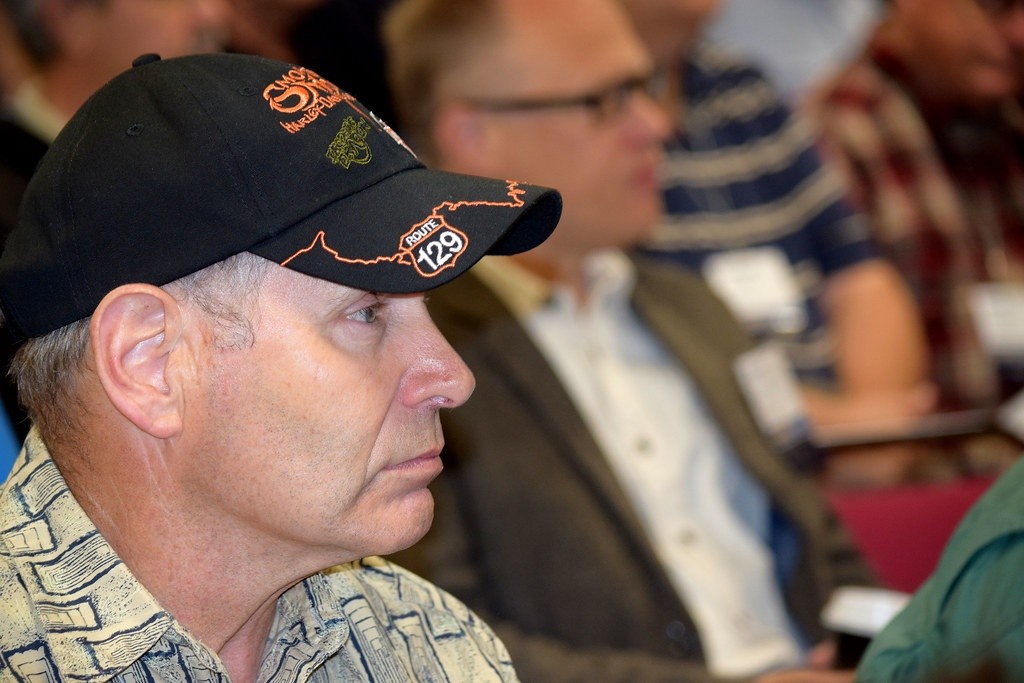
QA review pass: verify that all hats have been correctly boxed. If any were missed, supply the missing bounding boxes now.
[3,54,563,346]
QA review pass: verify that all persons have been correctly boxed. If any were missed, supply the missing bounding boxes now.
[0,1,1024,683]
[1,50,564,683]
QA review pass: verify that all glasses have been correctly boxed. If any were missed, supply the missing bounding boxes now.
[461,51,667,120]
